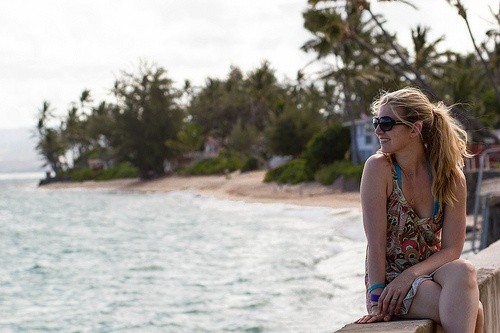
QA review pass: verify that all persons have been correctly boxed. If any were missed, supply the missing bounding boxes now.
[354,86,485,333]
[410,191,415,205]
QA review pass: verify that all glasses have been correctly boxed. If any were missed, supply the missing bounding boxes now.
[373,116,406,131]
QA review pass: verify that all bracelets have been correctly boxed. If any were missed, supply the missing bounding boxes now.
[368,283,385,309]
